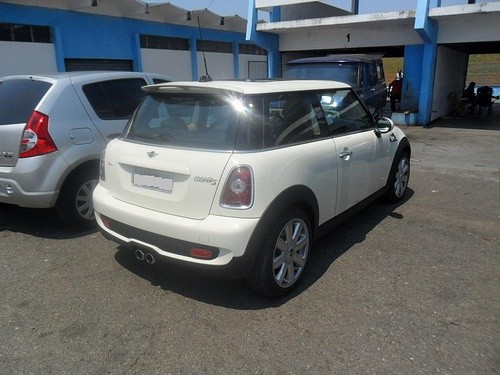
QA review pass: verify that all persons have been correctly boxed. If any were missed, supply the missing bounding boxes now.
[462,81,477,112]
[395,69,404,80]
[389,77,404,113]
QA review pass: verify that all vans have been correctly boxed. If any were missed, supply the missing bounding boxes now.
[280,53,389,121]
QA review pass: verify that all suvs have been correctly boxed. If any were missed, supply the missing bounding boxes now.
[0,70,171,232]
[91,16,412,299]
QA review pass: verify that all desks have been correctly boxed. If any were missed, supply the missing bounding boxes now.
[450,97,468,116]
